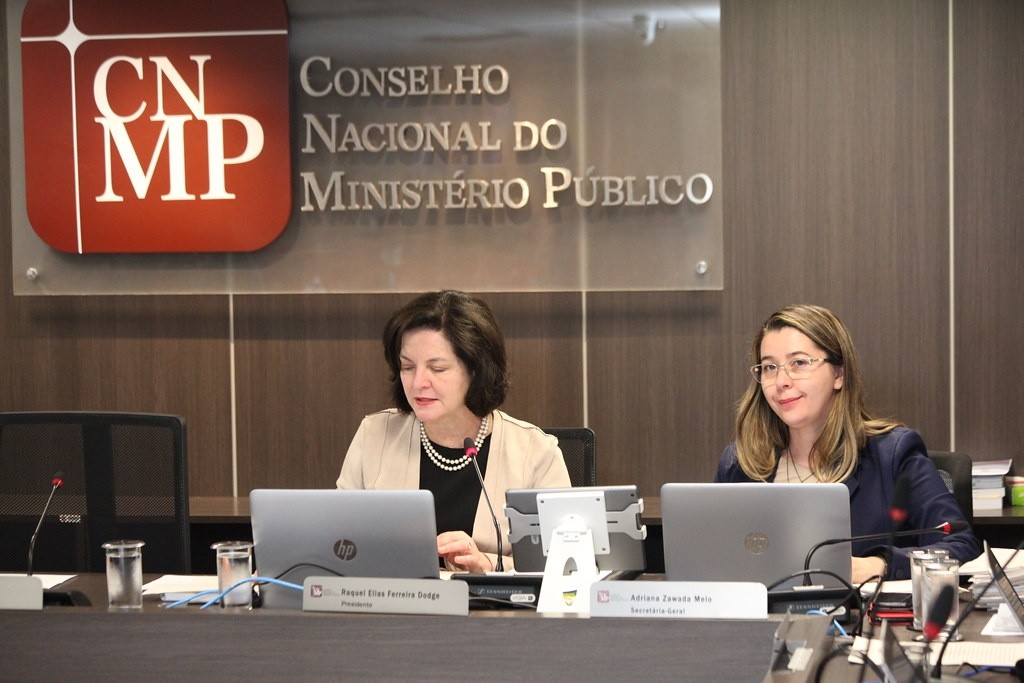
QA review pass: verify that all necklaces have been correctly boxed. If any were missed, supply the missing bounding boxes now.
[418,418,489,471]
[785,443,826,484]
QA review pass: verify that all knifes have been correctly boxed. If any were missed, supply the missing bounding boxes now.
[882,620,923,683]
[984,540,1024,634]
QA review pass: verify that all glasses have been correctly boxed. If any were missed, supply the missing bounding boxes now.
[750,358,839,384]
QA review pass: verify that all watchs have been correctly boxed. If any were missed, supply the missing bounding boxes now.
[879,548,894,575]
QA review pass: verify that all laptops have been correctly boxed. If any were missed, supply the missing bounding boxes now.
[249,488,441,611]
[878,618,926,683]
[660,483,852,588]
[984,539,1024,632]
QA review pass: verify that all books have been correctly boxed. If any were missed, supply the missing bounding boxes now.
[970,457,1014,511]
[958,547,1024,636]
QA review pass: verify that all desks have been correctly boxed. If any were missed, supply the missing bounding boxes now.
[0,573,1024,683]
[0,494,1024,575]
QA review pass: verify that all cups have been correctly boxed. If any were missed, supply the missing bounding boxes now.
[908,550,950,630]
[102,540,145,608]
[919,560,959,641]
[211,541,254,610]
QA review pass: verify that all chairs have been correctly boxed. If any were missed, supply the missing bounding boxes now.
[544,427,597,487]
[924,449,974,529]
[0,410,191,574]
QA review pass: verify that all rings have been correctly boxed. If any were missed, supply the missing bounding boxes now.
[468,542,470,547]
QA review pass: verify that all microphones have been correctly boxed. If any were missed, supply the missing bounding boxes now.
[451,437,544,611]
[25,469,92,607]
[766,510,971,683]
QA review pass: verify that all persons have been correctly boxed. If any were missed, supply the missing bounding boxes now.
[332,289,573,574]
[713,305,983,586]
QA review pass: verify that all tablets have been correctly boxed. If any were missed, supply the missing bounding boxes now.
[505,485,647,571]
